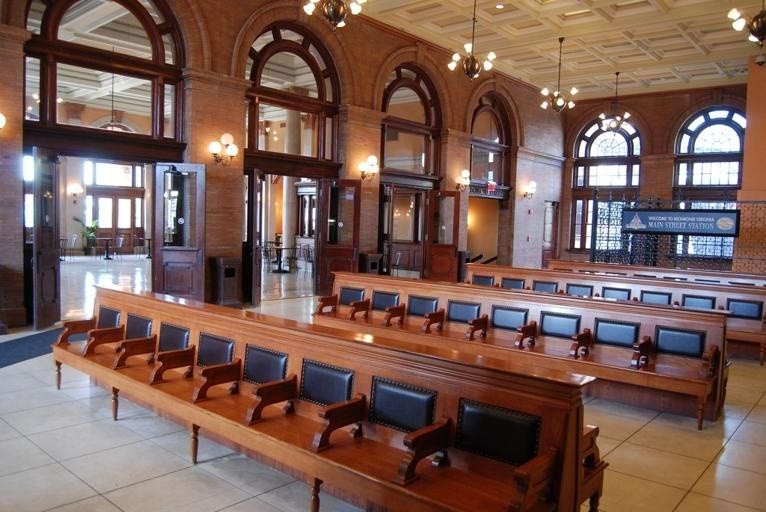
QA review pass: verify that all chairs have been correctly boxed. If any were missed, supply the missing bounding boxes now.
[89,238,101,259]
[132,234,146,260]
[66,235,78,257]
[391,251,402,276]
[109,233,125,259]
[261,239,316,277]
[60,238,68,260]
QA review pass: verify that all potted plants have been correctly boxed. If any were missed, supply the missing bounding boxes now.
[72,216,99,256]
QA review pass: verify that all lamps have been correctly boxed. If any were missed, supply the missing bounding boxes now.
[598,69,633,134]
[442,2,498,84]
[539,37,580,115]
[208,132,240,166]
[358,154,380,181]
[67,180,84,207]
[726,1,765,66]
[297,1,369,30]
[454,168,472,191]
[522,180,538,200]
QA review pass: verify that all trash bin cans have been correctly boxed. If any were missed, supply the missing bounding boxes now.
[458,251,473,282]
[359,254,381,276]
[217,257,244,309]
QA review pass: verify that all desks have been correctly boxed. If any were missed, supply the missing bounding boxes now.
[144,238,153,258]
[96,237,113,261]
